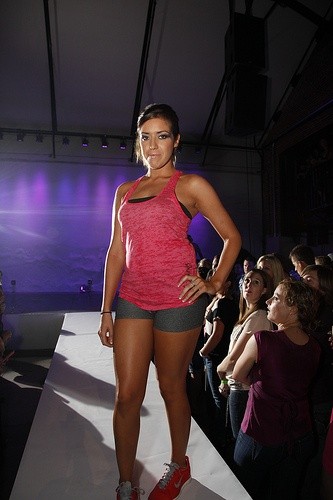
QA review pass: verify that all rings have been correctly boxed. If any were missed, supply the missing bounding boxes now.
[191,281,195,285]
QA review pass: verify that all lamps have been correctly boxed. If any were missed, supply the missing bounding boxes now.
[36,135,43,142]
[82,137,88,147]
[17,132,24,142]
[120,139,127,150]
[102,138,108,148]
[81,285,90,293]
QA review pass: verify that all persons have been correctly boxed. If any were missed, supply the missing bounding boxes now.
[195,245,333,500]
[98,103,243,500]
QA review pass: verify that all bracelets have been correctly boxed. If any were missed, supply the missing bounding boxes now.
[100,312,112,314]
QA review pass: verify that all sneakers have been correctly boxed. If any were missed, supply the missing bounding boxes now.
[114,480,144,500]
[148,456,192,500]
